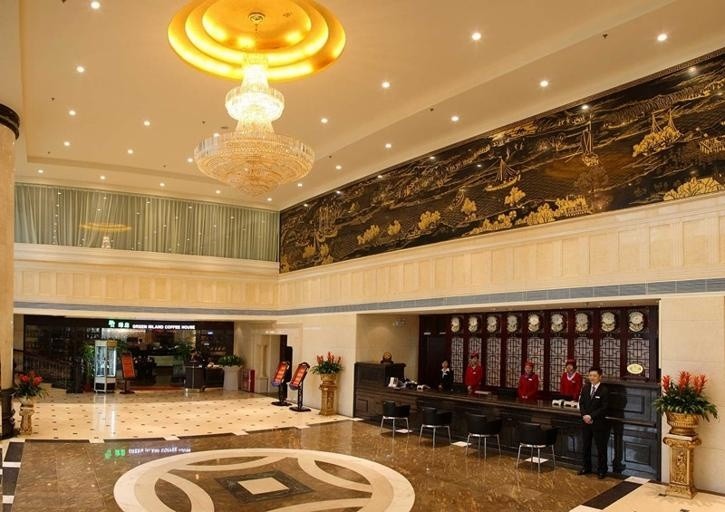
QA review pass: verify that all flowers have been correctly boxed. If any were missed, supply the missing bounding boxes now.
[649,371,720,425]
[216,354,244,366]
[13,369,53,402]
[308,350,345,375]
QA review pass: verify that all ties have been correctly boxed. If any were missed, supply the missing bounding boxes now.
[590,386,597,401]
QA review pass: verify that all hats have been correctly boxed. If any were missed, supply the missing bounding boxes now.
[471,352,479,357]
[567,359,576,363]
[525,361,535,366]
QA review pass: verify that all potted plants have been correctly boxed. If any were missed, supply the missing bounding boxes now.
[82,342,95,392]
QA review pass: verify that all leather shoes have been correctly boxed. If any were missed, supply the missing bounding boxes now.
[598,473,607,479]
[577,469,592,475]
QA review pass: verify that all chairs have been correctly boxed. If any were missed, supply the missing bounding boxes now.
[380,400,411,439]
[513,421,560,473]
[417,406,453,450]
[463,410,505,461]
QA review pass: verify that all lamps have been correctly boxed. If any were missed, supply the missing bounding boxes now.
[192,11,316,199]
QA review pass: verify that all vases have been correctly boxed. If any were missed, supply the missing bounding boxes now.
[320,373,337,386]
[664,411,701,437]
[218,365,244,391]
[14,394,37,407]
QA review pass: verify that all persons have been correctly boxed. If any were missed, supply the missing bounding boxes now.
[464,351,483,392]
[560,359,584,404]
[518,360,539,402]
[577,367,613,479]
[437,358,454,394]
